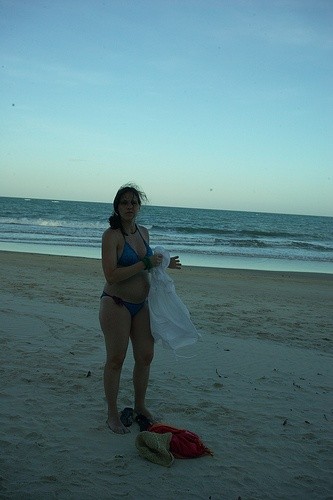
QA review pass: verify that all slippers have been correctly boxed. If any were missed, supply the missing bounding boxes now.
[135,414,151,432]
[120,407,134,427]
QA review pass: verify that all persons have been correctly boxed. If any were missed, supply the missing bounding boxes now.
[98,187,182,435]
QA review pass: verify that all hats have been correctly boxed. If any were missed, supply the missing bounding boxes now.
[135,431,174,467]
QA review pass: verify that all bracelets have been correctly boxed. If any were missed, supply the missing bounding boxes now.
[139,256,153,271]
